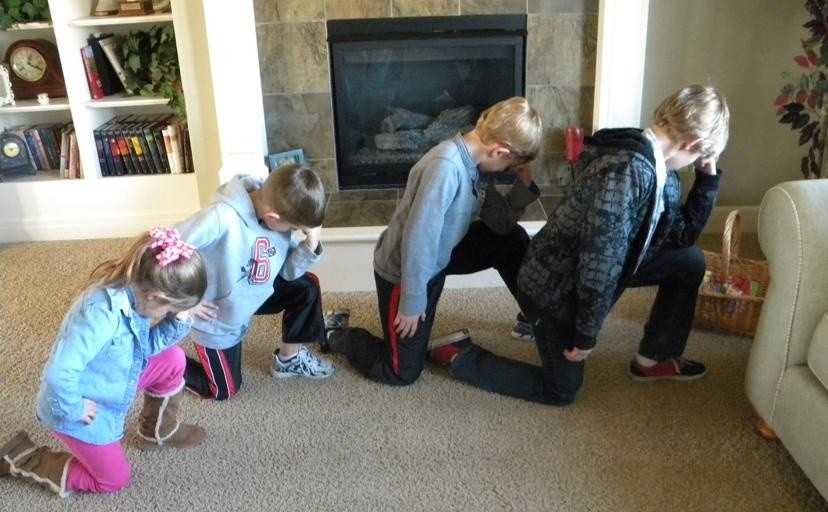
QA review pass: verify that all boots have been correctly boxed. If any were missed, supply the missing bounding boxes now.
[136,377,208,450]
[0,430,75,497]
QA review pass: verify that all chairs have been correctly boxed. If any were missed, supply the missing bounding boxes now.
[744,178,828,502]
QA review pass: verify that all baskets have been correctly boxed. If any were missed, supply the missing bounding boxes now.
[693,210,774,337]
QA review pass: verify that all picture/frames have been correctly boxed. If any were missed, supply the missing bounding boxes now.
[267,148,305,173]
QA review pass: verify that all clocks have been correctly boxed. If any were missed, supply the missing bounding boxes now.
[2,141,21,158]
[5,40,63,100]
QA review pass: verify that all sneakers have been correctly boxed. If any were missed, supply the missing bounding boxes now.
[629,355,706,382]
[427,326,471,367]
[509,312,536,342]
[322,308,350,332]
[271,347,337,380]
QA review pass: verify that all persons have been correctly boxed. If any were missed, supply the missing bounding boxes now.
[312,94,543,388]
[423,82,731,408]
[1,226,207,498]
[156,162,334,402]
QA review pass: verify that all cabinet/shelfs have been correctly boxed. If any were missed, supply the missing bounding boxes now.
[0,0,217,243]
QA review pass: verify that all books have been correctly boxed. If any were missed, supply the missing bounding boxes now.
[6,34,188,178]
